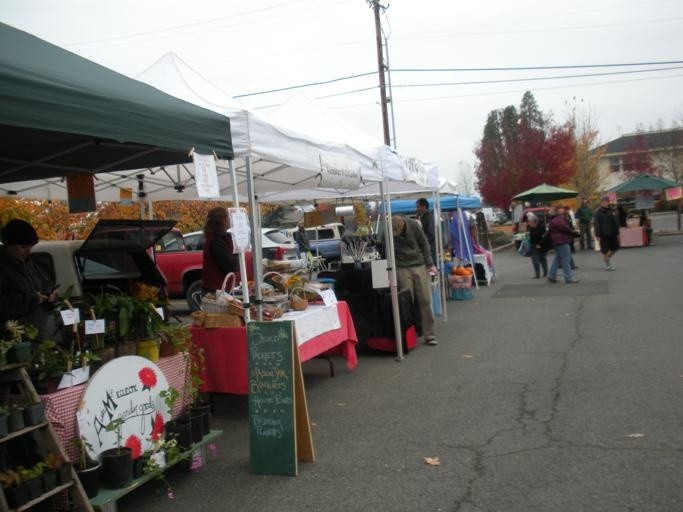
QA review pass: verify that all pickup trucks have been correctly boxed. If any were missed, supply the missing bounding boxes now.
[508,204,575,253]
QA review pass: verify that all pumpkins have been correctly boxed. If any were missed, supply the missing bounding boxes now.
[453,266,472,275]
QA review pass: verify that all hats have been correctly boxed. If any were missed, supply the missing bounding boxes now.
[1,219,38,246]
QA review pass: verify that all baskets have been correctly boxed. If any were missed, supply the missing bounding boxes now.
[191,273,320,328]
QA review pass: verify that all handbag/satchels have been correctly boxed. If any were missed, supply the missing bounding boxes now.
[518,236,532,257]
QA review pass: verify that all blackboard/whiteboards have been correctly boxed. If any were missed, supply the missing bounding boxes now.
[245,320,296,476]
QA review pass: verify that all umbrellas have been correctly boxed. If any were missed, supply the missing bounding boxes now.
[510,182,580,225]
[603,172,681,194]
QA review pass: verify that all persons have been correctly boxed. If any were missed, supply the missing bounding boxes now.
[200,206,240,298]
[391,216,439,346]
[294,223,312,268]
[0,218,65,354]
[415,197,436,267]
[643,213,654,246]
[525,196,621,284]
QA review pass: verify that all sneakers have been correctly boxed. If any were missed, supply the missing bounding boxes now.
[548,277,559,283]
[567,276,580,283]
[604,265,616,271]
[425,338,438,345]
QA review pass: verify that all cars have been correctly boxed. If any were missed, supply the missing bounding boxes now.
[23,214,178,330]
[143,219,346,319]
[491,212,509,224]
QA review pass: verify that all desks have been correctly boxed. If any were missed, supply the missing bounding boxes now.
[169,300,347,415]
